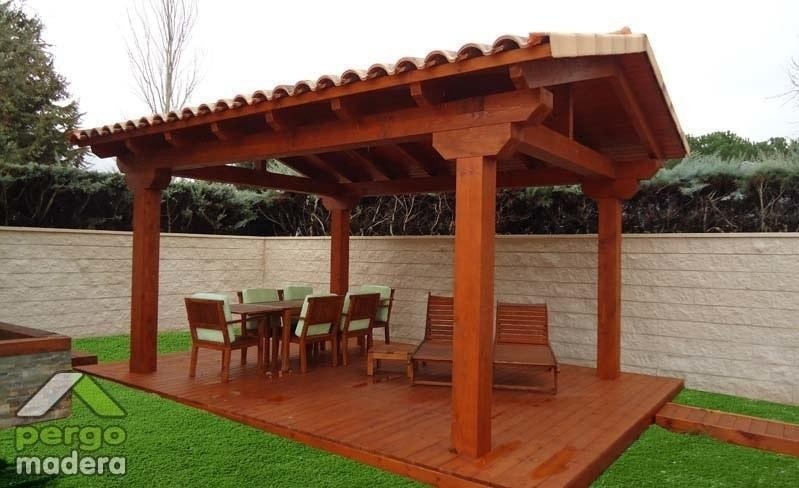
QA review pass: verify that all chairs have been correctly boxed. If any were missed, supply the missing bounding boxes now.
[408,291,454,385]
[184,283,395,383]
[491,298,561,396]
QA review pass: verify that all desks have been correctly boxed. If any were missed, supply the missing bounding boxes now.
[367,343,418,378]
